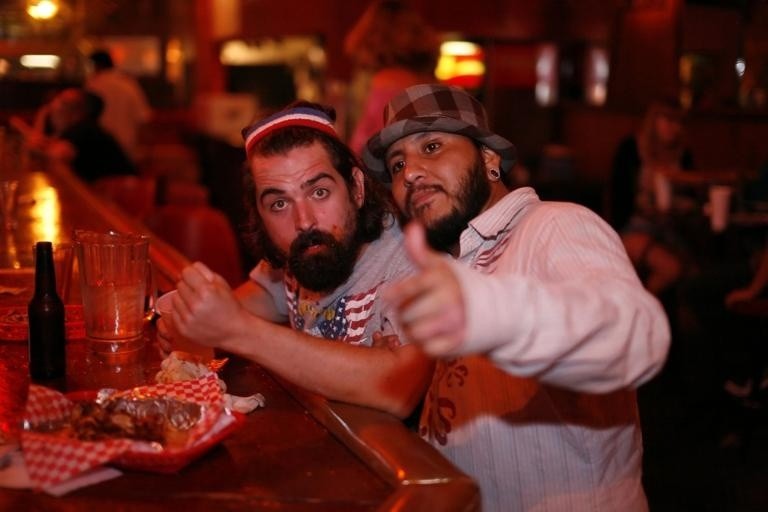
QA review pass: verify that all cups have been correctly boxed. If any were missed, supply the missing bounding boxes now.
[707,184,733,234]
[69,228,156,357]
[155,290,216,365]
[30,243,74,306]
[654,172,674,212]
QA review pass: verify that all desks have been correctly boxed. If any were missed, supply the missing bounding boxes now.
[1,113,476,512]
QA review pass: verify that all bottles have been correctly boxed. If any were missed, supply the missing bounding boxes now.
[26,241,69,393]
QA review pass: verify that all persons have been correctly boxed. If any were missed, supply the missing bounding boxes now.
[366,84,673,512]
[8,50,766,447]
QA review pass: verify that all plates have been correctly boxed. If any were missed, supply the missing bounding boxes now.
[0,302,86,344]
[0,389,249,477]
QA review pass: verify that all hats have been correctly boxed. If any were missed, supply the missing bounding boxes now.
[361,84,516,187]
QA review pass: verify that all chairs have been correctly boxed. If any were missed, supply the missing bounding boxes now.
[146,207,242,283]
[91,176,158,218]
